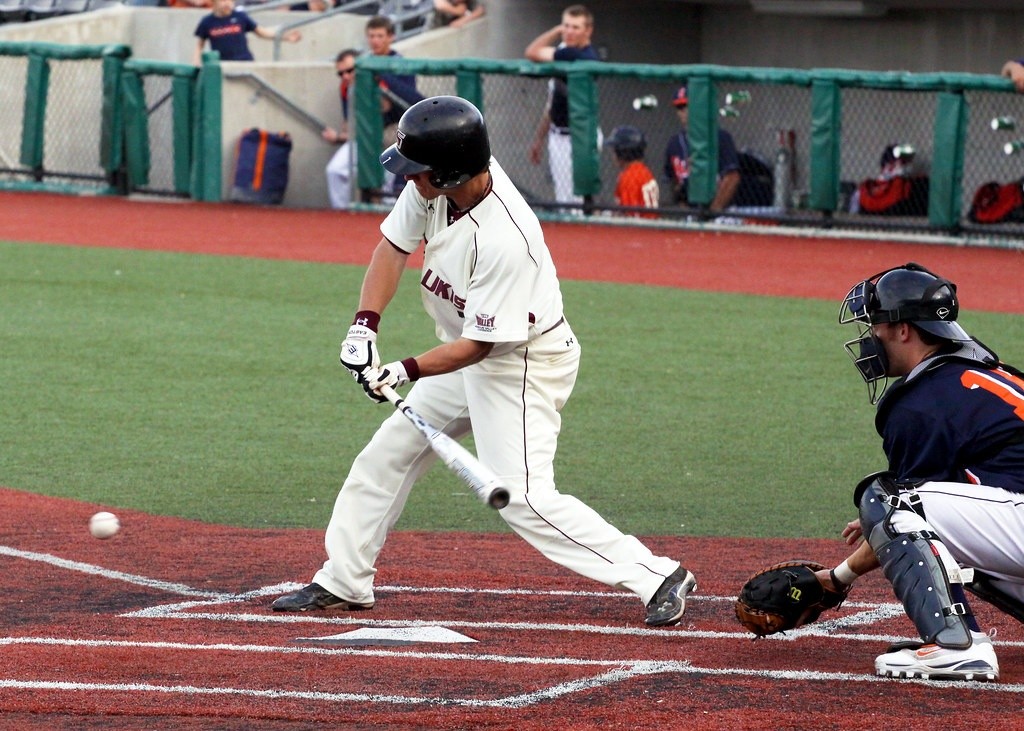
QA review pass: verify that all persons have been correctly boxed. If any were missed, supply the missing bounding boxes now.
[272,96,698,626]
[277,0,340,13]
[660,86,740,224]
[603,124,662,220]
[321,15,428,209]
[525,5,604,216]
[850,144,915,217]
[429,0,485,30]
[193,0,300,66]
[157,0,213,8]
[1002,56,1024,90]
[734,263,1024,678]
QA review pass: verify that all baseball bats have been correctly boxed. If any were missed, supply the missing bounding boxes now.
[380,381,511,512]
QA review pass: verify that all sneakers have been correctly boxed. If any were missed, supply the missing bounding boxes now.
[874,628,1001,682]
[270,582,375,612]
[643,567,697,625]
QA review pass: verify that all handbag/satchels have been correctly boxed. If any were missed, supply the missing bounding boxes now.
[856,173,927,216]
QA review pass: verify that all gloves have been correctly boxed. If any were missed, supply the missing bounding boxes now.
[339,324,410,404]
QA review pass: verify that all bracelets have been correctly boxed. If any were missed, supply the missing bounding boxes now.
[832,560,857,585]
[336,129,339,141]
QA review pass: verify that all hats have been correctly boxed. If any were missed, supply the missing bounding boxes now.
[669,87,687,106]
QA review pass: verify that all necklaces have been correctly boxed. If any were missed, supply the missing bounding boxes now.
[446,172,491,223]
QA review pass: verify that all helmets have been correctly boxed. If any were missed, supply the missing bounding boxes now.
[871,268,975,343]
[378,95,490,190]
[604,124,646,151]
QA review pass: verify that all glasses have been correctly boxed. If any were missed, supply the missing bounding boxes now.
[337,67,355,77]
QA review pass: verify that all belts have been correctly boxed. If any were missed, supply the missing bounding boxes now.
[549,124,571,135]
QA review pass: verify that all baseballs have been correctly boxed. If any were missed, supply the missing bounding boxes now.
[88,511,120,540]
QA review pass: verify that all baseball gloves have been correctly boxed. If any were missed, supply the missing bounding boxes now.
[731,559,844,635]
